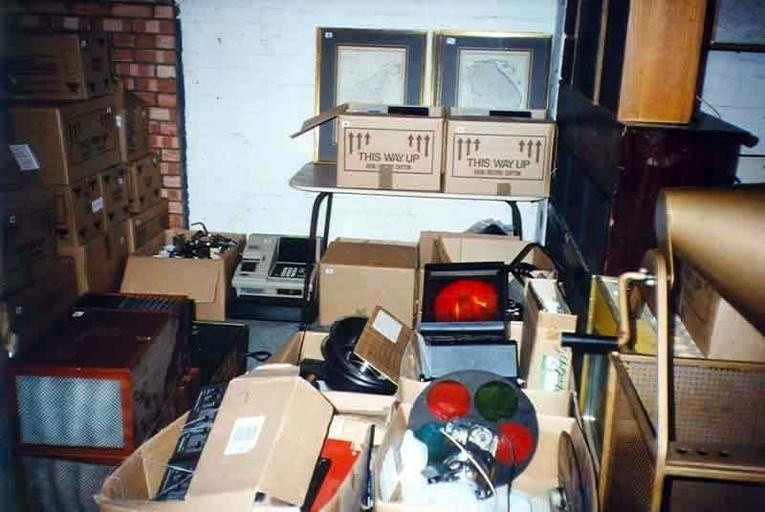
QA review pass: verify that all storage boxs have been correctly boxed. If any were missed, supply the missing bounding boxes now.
[291,102,446,191]
[559,0,708,125]
[441,106,560,198]
[670,249,765,365]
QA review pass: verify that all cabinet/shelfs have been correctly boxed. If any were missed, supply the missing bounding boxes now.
[544,78,759,336]
[578,273,706,483]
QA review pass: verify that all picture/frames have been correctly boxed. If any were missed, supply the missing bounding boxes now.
[430,30,554,175]
[312,27,426,168]
